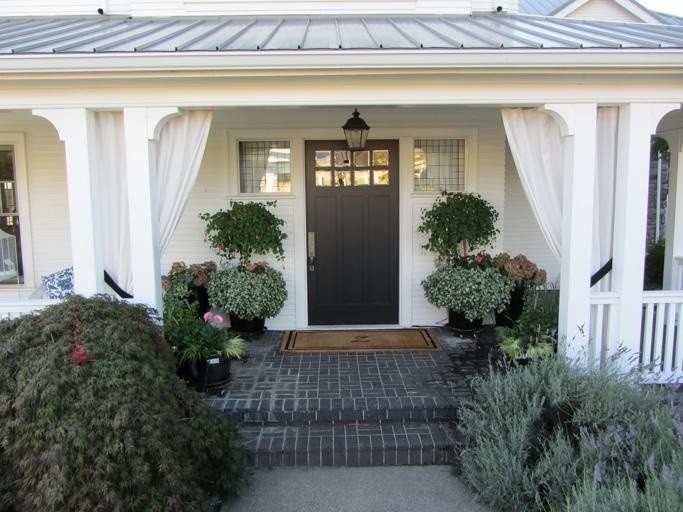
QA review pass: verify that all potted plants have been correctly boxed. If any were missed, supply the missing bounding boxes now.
[414,183,516,340]
[497,303,558,369]
[194,193,290,342]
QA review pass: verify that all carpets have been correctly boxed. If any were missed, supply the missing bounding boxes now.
[276,325,443,355]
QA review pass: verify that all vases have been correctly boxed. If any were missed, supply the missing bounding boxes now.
[498,280,526,327]
[186,282,211,316]
[158,259,214,300]
[184,352,235,384]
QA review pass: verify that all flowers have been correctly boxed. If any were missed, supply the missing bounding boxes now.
[488,247,547,288]
[202,262,290,323]
[417,247,517,323]
[173,309,247,369]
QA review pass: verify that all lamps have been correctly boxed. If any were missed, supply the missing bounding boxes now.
[340,107,370,153]
